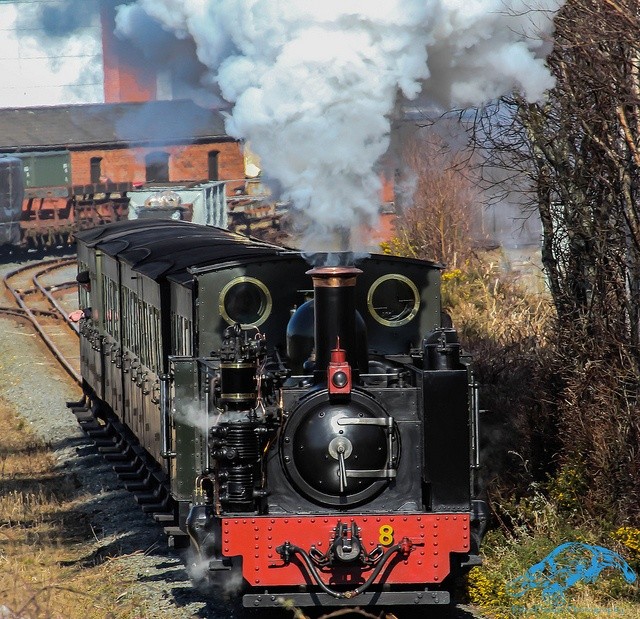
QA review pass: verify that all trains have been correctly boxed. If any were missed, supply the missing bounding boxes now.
[65,215,497,619]
[0,156,290,262]
[124,178,233,238]
